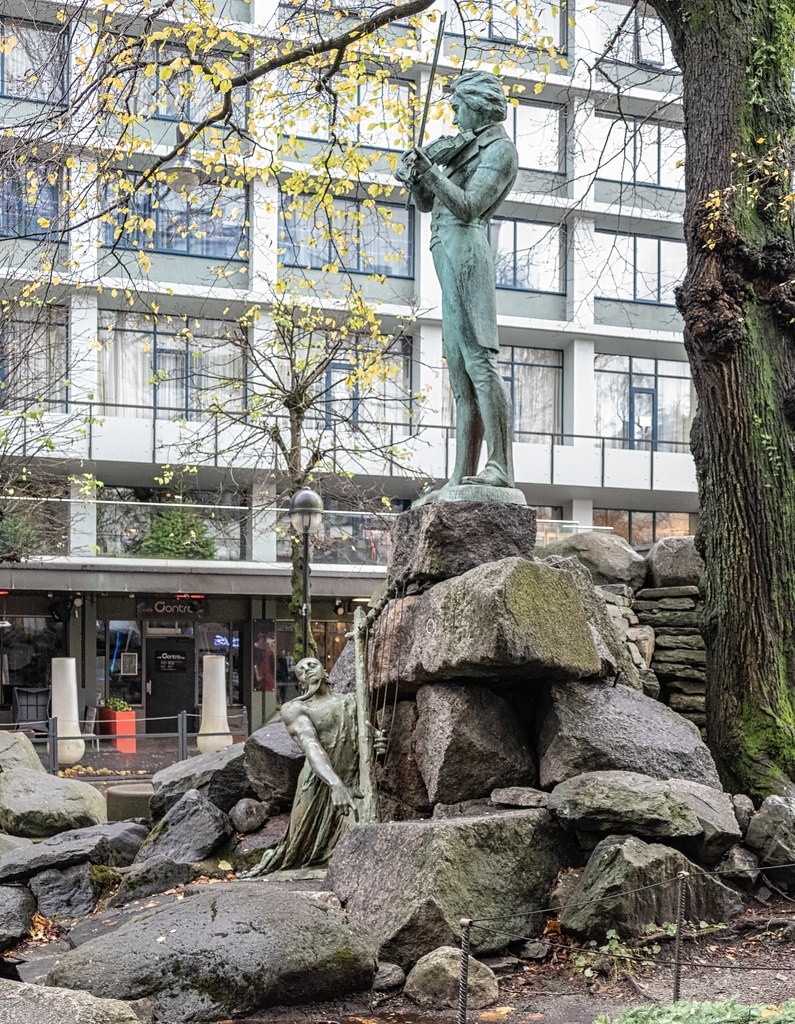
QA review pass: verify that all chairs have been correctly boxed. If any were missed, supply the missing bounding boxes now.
[82,706,98,749]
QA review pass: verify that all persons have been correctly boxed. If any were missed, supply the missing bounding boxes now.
[281,656,386,865]
[397,71,520,488]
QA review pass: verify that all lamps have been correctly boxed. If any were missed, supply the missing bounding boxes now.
[72,596,83,607]
[333,604,345,616]
[47,600,70,624]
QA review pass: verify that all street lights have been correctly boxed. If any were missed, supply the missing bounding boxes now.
[287,485,324,659]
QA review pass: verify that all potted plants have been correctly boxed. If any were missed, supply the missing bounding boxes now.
[98,696,136,753]
